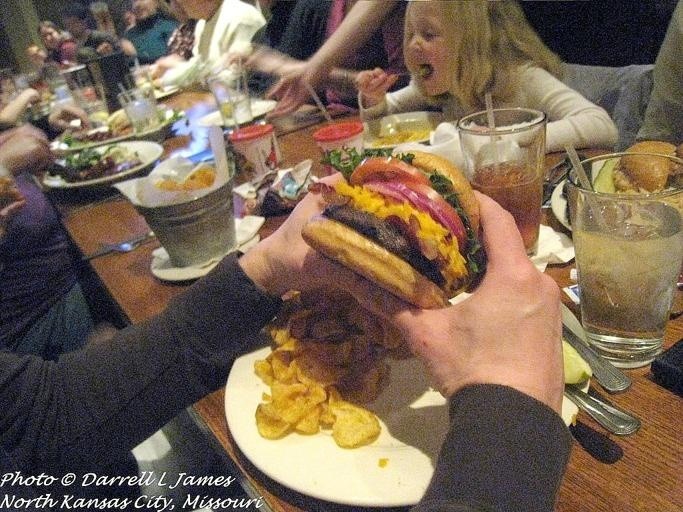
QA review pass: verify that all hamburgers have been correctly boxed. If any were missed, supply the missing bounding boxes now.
[301,145,485,310]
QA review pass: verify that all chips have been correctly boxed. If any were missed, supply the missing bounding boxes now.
[253,297,416,449]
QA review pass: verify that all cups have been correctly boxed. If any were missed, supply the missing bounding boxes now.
[311,120,366,176]
[454,105,547,262]
[563,151,680,369]
[229,125,284,182]
[63,65,101,103]
[126,68,157,102]
[206,72,251,123]
[115,87,160,135]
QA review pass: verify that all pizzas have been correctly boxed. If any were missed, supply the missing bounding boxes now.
[615,140,676,193]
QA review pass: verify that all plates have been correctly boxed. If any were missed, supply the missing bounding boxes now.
[362,107,444,151]
[51,105,183,152]
[141,81,182,97]
[41,140,163,188]
[197,98,277,128]
[223,289,595,510]
[149,217,261,284]
[551,154,678,233]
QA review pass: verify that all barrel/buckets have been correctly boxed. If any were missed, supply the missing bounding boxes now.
[58,64,99,106]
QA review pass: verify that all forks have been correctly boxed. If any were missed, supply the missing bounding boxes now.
[105,235,148,254]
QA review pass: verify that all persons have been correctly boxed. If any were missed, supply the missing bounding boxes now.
[1,0,425,362]
[0,170,574,512]
[353,0,620,153]
[634,0,682,145]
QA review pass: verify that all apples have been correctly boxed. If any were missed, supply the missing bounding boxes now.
[563,341,592,387]
